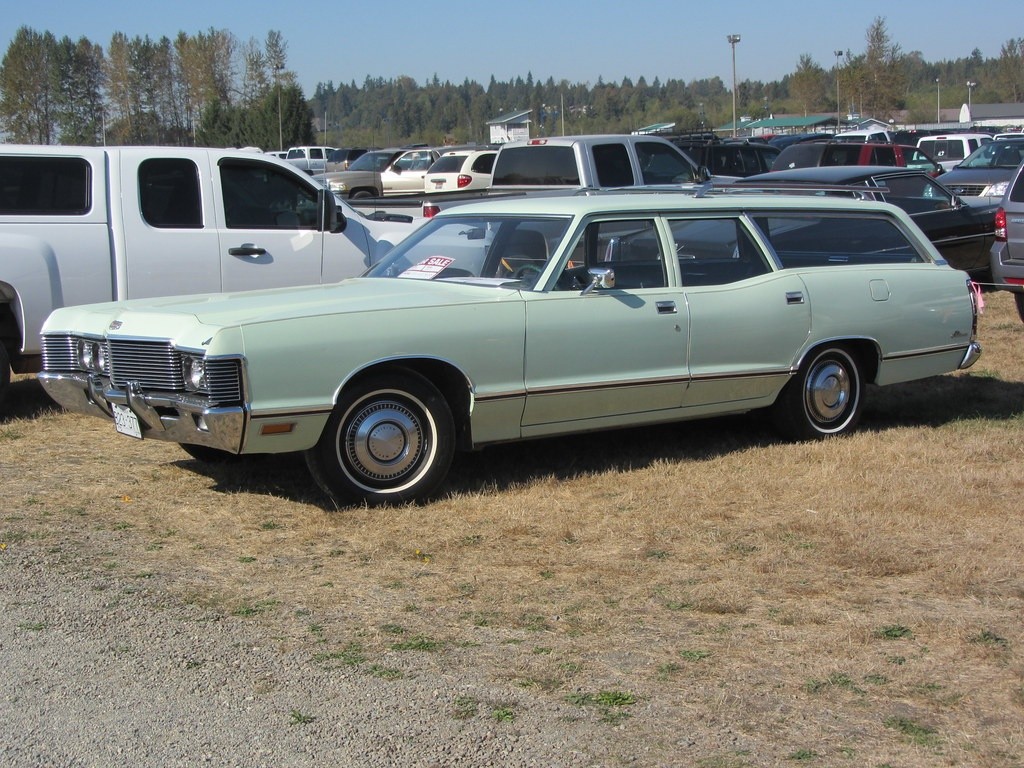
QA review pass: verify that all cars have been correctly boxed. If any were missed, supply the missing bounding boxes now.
[924,138,1024,209]
[422,149,499,192]
[623,166,1007,282]
[380,146,463,193]
[34,183,984,509]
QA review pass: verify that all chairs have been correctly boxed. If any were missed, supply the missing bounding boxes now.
[246,172,298,225]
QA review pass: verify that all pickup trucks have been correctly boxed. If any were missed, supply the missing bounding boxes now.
[283,146,339,179]
[345,134,747,247]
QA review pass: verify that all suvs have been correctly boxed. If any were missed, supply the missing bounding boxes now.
[770,138,949,181]
[329,145,369,174]
[298,148,424,224]
[653,124,954,177]
[990,157,1024,322]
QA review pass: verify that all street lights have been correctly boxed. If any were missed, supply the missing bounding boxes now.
[966,80,977,113]
[936,77,941,125]
[728,34,743,139]
[834,50,845,134]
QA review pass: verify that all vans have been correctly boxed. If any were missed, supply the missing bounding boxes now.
[910,133,995,174]
[0,143,497,405]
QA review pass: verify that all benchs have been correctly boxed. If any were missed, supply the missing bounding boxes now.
[726,251,916,280]
[519,259,692,291]
